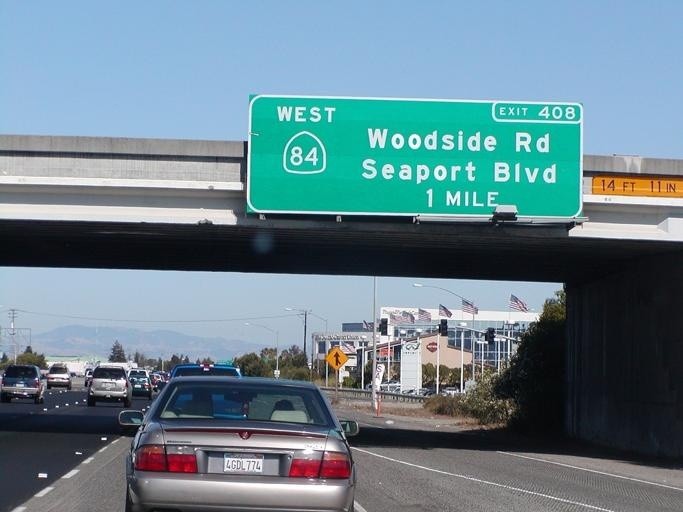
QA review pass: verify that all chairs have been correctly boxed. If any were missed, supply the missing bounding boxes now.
[178,400,308,426]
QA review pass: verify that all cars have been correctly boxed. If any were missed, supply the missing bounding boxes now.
[84,370,93,387]
[168,363,249,419]
[2,365,44,404]
[46,363,71,390]
[85,368,92,375]
[366,384,459,397]
[128,368,167,400]
[87,365,133,408]
[117,377,359,512]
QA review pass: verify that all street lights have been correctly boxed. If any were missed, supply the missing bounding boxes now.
[244,322,281,379]
[412,283,474,381]
[285,308,306,354]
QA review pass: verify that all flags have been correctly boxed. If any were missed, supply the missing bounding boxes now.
[391,313,403,326]
[462,298,478,314]
[510,294,528,313]
[439,304,452,319]
[402,311,414,324]
[419,308,431,322]
